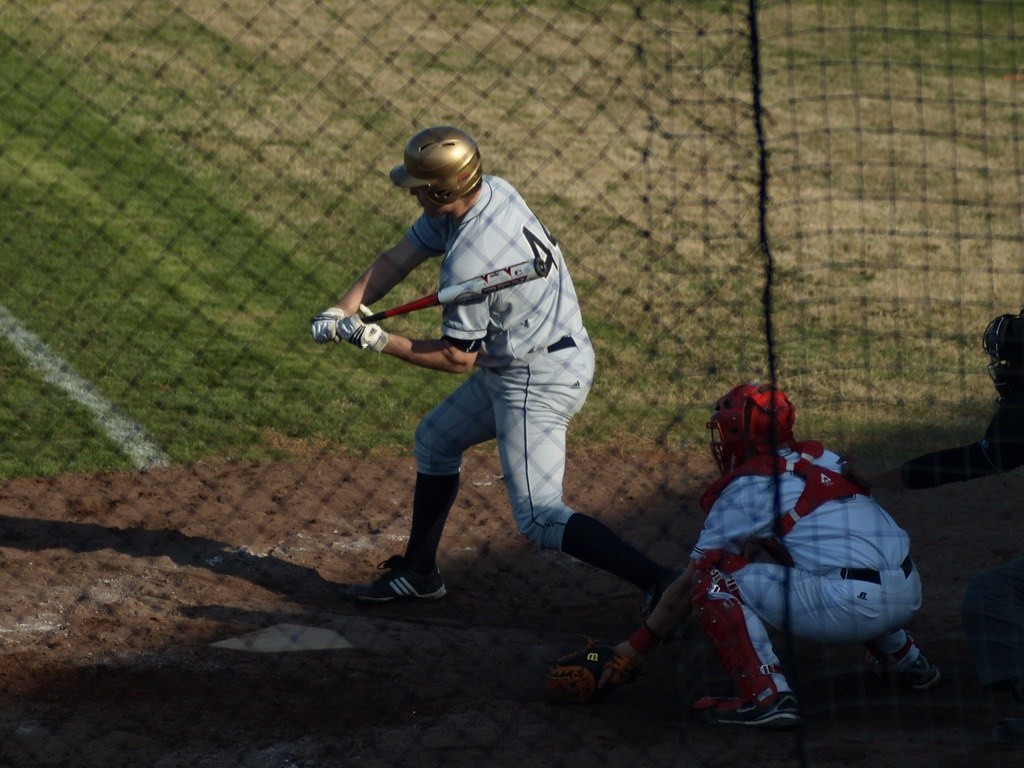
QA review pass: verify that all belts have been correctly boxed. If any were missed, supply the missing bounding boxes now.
[546,333,577,353]
[840,555,913,586]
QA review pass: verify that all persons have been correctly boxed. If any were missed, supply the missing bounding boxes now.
[312,128,683,624]
[539,383,940,727]
[879,309,1024,491]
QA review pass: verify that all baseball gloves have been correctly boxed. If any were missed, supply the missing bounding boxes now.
[537,634,638,703]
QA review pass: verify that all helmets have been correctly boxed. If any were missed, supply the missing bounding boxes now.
[705,382,796,475]
[981,310,1024,409]
[390,126,483,206]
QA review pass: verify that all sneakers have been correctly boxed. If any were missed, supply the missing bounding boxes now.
[870,649,940,690]
[346,554,449,604]
[700,690,804,732]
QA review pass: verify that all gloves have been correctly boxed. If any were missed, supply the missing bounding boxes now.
[310,305,354,343]
[338,301,392,354]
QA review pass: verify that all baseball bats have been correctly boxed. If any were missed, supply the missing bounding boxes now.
[358,257,548,325]
